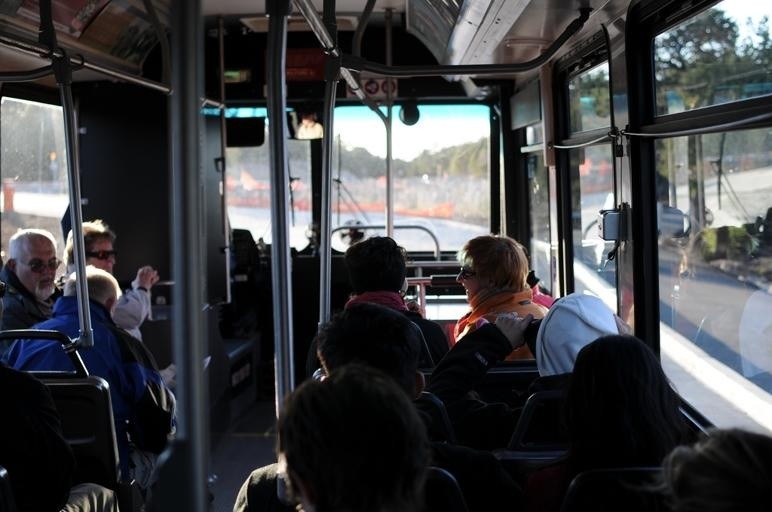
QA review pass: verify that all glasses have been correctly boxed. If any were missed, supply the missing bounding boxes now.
[458,267,479,279]
[86,249,115,260]
[16,257,62,273]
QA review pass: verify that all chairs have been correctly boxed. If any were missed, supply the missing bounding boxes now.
[331,294,709,512]
[0,227,262,509]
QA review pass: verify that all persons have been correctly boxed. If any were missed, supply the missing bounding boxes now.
[230,229,772,512]
[1,221,174,512]
